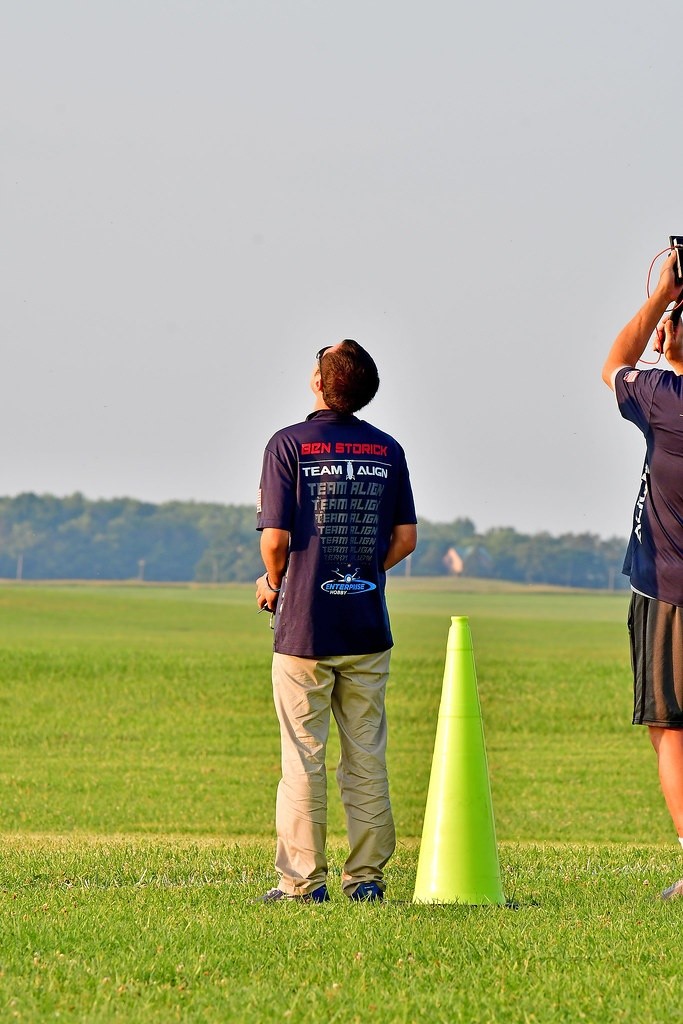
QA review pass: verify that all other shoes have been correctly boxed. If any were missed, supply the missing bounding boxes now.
[661,880,683,899]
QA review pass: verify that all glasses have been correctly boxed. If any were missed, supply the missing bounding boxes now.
[316,345,332,387]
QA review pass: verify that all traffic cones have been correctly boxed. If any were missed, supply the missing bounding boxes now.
[413,616,509,908]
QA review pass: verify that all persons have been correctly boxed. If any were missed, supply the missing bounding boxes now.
[601,246,683,893]
[249,331,420,904]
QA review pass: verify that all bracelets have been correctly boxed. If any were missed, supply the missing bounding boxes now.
[265,572,281,592]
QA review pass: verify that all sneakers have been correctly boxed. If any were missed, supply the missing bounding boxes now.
[349,881,383,902]
[261,884,331,904]
